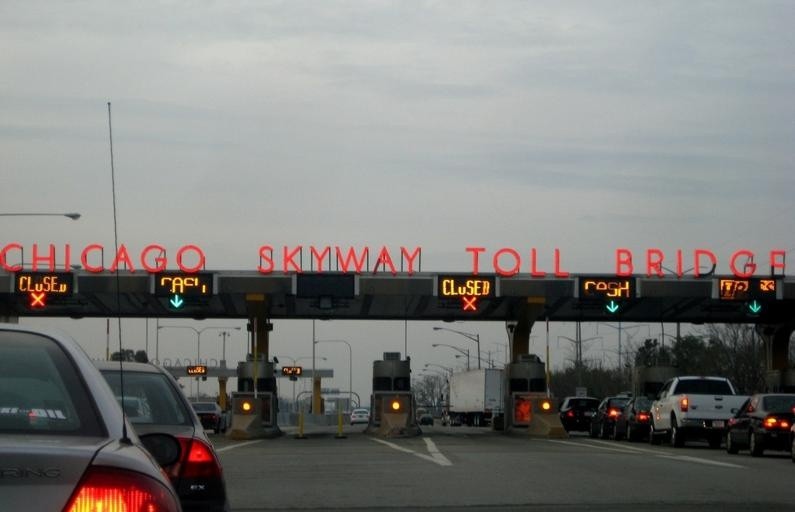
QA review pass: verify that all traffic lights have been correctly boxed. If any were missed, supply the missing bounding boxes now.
[319,294,333,309]
[603,297,622,316]
[231,398,261,416]
[25,291,47,311]
[532,398,557,415]
[462,296,479,313]
[382,395,412,414]
[168,293,184,310]
[745,298,762,317]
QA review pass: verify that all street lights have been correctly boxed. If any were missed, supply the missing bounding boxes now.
[311,339,353,410]
[0,211,85,220]
[275,354,330,408]
[416,322,642,383]
[155,321,244,399]
[648,263,711,341]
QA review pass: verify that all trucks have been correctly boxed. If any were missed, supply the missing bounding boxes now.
[628,363,680,403]
[434,367,506,428]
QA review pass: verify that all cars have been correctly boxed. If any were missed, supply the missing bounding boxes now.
[110,393,151,419]
[91,354,231,512]
[787,424,795,464]
[418,414,435,427]
[0,318,184,512]
[187,400,227,438]
[349,407,369,426]
[415,407,427,418]
[325,409,333,416]
[716,389,795,459]
[556,393,655,449]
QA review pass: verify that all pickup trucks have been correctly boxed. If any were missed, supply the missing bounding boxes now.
[647,374,754,449]
